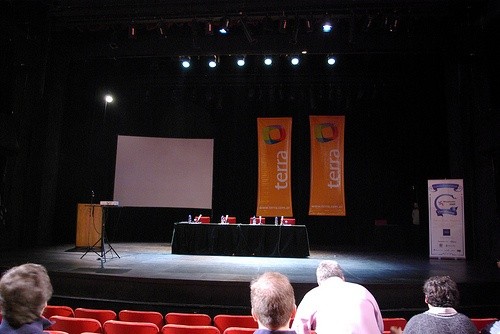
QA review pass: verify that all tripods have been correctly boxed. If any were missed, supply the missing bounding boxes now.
[80,204,121,262]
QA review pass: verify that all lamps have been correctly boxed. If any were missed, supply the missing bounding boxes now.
[127,14,401,37]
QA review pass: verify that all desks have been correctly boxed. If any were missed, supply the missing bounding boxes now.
[171,222,310,258]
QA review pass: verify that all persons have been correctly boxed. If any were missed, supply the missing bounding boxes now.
[249,272,296,334]
[0,263,53,334]
[402,276,478,334]
[291,260,384,334]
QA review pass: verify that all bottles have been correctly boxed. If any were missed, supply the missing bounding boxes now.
[253,215,256,224]
[188,215,191,223]
[221,214,224,223]
[275,216,278,225]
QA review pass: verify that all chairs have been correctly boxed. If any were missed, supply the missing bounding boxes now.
[283,218,296,225]
[195,216,210,222]
[224,217,237,223]
[249,217,266,224]
[0,306,498,334]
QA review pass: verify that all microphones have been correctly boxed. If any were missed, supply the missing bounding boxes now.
[225,215,229,223]
[92,191,94,197]
[198,215,202,220]
[280,216,284,225]
[258,216,263,225]
